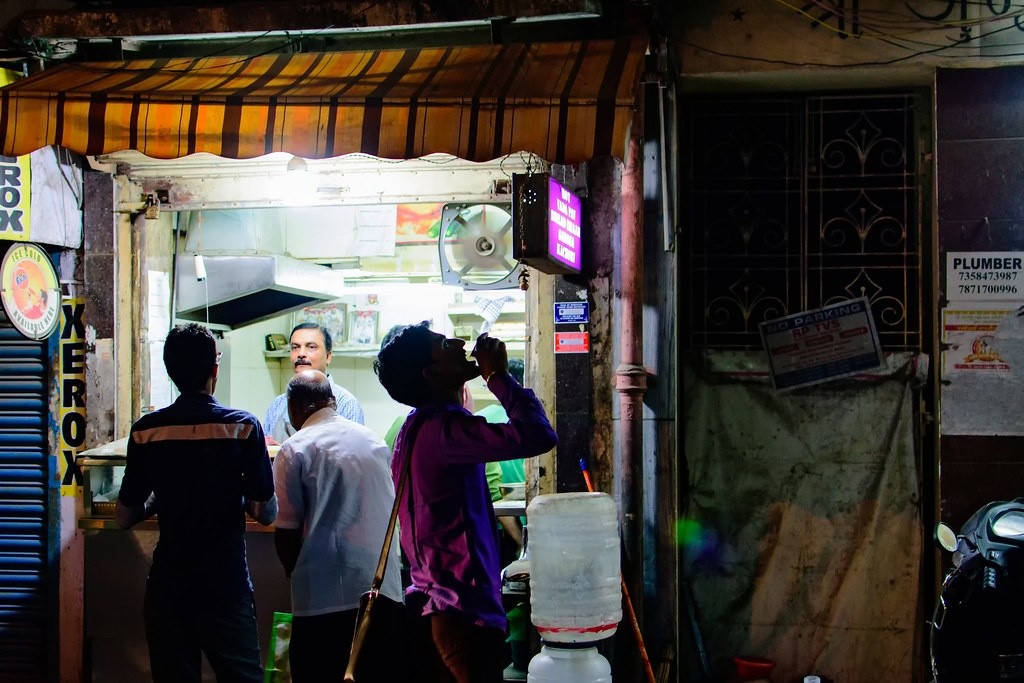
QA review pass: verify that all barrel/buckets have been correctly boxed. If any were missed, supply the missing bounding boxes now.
[525,491,623,643]
[526,637,612,683]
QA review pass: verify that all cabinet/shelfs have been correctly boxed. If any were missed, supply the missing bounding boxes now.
[264,270,528,358]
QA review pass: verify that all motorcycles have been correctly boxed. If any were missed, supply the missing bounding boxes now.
[930,496,1024,683]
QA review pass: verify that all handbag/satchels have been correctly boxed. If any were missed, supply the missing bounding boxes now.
[343,591,427,682]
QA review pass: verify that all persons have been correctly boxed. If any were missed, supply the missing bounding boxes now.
[372,326,557,683]
[263,323,364,445]
[115,323,278,683]
[271,370,408,683]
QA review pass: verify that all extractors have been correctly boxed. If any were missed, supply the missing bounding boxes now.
[174,208,344,332]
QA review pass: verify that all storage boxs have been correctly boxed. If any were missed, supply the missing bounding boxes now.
[266,334,288,352]
